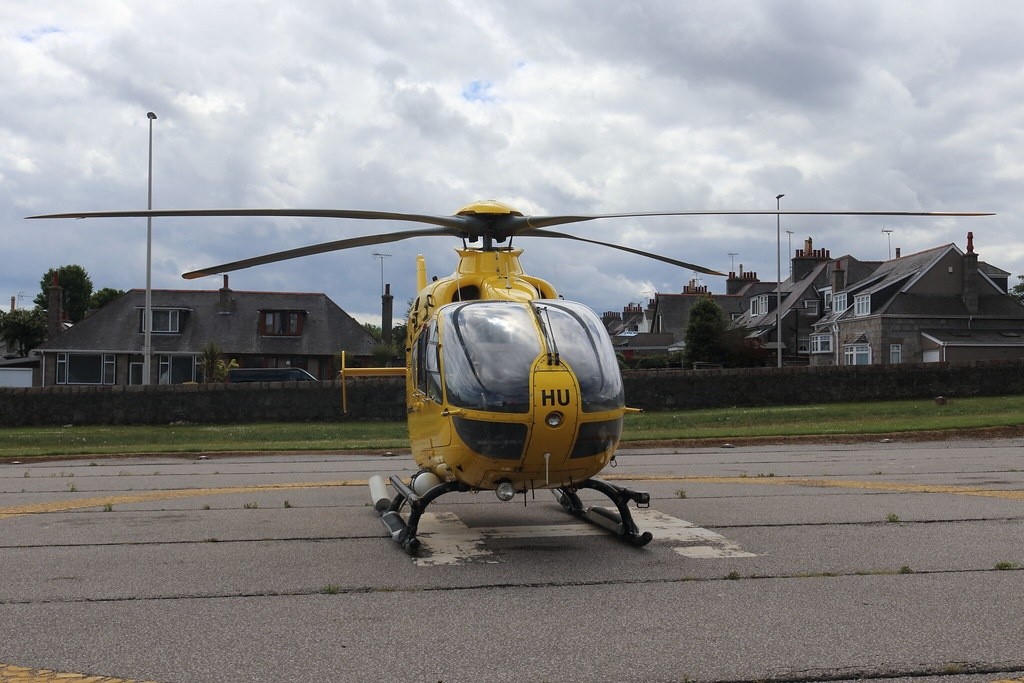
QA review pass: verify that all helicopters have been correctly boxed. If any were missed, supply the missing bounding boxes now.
[23,199,997,556]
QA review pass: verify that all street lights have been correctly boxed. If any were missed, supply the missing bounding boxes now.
[143,111,157,383]
[776,193,785,368]
